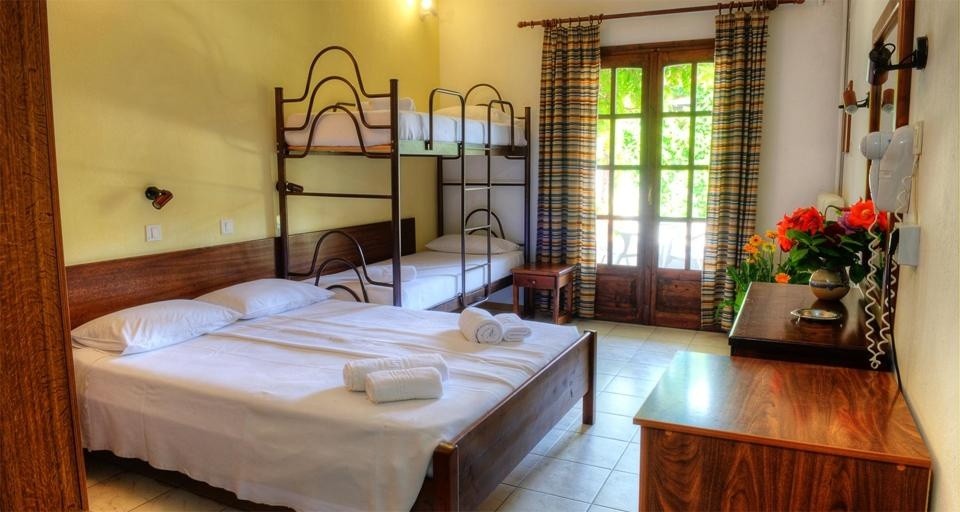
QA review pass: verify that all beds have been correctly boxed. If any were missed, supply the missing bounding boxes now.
[274,46,531,155]
[69,278,597,512]
[281,249,530,312]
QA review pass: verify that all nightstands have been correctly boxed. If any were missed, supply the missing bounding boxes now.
[510,263,577,325]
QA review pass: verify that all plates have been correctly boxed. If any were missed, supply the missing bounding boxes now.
[790,307,844,321]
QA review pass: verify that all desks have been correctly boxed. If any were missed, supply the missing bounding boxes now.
[662,233,704,267]
[728,282,893,371]
[616,231,638,266]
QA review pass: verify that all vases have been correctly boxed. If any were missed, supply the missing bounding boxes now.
[809,271,851,302]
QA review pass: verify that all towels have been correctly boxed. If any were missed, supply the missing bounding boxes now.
[368,99,412,110]
[360,265,416,284]
[459,307,505,345]
[343,353,448,392]
[493,312,532,343]
[355,98,369,108]
[365,367,443,402]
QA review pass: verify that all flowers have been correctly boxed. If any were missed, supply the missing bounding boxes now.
[776,194,899,292]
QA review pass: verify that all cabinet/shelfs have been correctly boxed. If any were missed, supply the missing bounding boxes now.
[633,350,932,512]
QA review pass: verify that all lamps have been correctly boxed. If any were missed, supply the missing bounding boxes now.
[276,183,304,192]
[865,36,930,86]
[145,186,173,210]
[842,81,871,115]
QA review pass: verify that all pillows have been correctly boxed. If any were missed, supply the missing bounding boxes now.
[69,299,244,356]
[425,234,520,253]
[432,105,522,125]
[193,278,336,319]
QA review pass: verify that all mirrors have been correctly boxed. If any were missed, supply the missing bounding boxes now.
[865,0,916,200]
